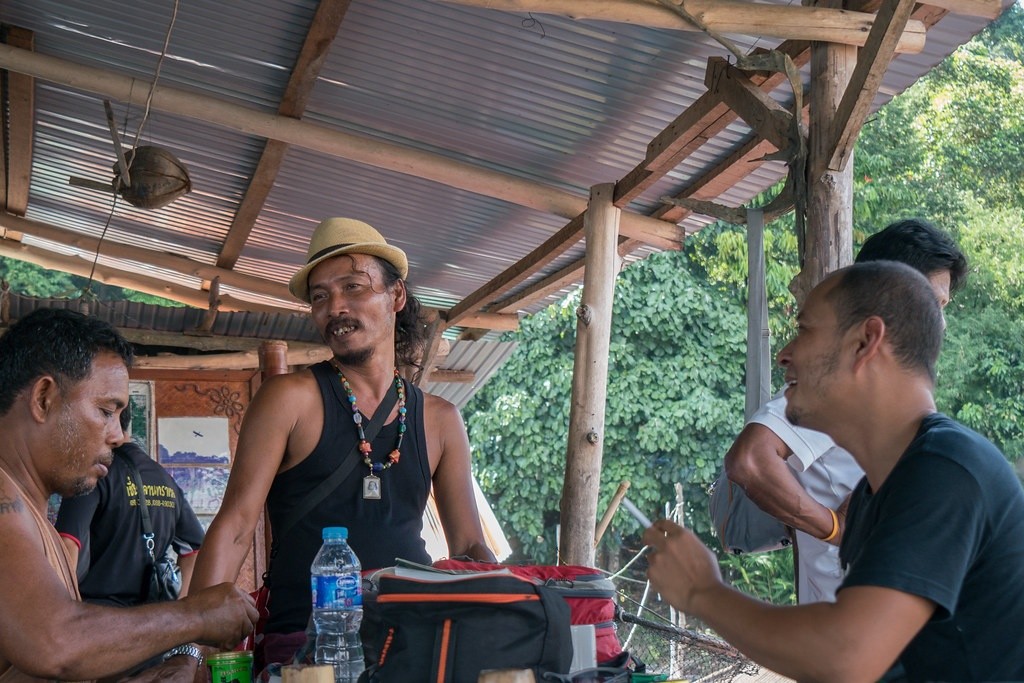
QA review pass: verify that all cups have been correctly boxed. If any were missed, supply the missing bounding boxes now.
[206,650,254,683]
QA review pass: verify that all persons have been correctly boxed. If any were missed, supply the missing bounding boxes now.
[644,261,1024,683]
[0,307,261,683]
[120,217,498,683]
[724,219,967,606]
[54,401,205,608]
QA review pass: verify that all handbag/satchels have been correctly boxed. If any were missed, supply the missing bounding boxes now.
[710,470,793,554]
[361,554,646,683]
[147,558,182,602]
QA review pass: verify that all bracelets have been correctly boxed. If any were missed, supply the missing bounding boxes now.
[163,645,203,665]
[821,508,839,541]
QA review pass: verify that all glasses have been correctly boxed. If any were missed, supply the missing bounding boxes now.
[543,667,628,683]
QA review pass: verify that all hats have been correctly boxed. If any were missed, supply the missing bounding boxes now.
[289,218,408,303]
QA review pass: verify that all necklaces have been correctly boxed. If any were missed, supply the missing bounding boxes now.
[332,358,407,499]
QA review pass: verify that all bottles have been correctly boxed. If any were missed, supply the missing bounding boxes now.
[311,526,366,683]
